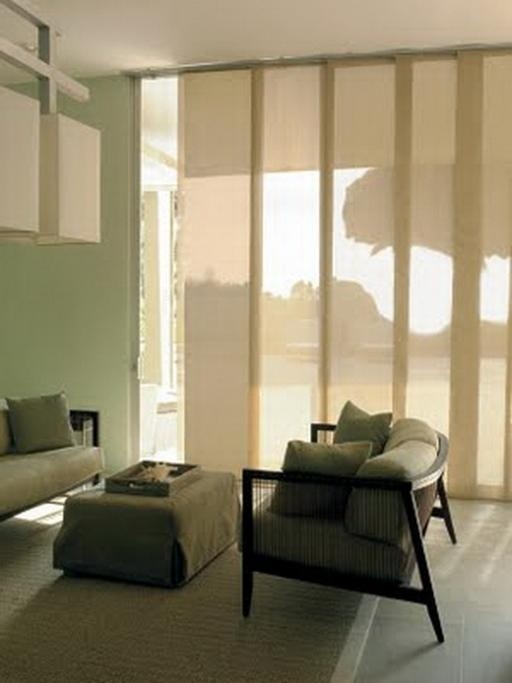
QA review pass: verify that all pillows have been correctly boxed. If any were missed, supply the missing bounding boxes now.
[266,441,372,518]
[333,401,393,458]
[383,418,441,451]
[8,391,76,452]
[0,398,12,455]
[343,438,439,546]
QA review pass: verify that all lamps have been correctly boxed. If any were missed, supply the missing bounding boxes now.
[38,78,103,246]
[0,85,40,240]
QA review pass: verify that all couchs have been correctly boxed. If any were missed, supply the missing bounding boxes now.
[243,424,457,643]
[0,410,104,524]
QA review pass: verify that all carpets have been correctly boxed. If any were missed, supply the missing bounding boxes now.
[0,474,380,683]
